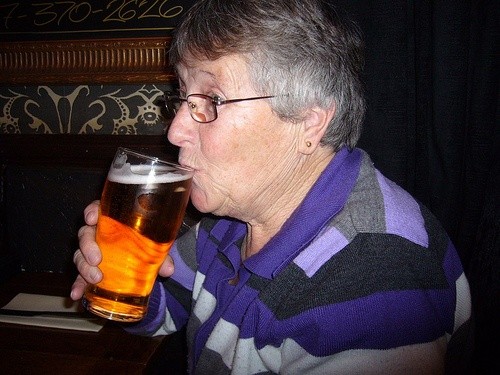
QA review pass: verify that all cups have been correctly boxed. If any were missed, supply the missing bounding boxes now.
[81,147,195,322]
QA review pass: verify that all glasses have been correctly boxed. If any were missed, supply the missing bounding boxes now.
[161,90,291,124]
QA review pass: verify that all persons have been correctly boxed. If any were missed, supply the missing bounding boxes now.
[71,0,474,375]
